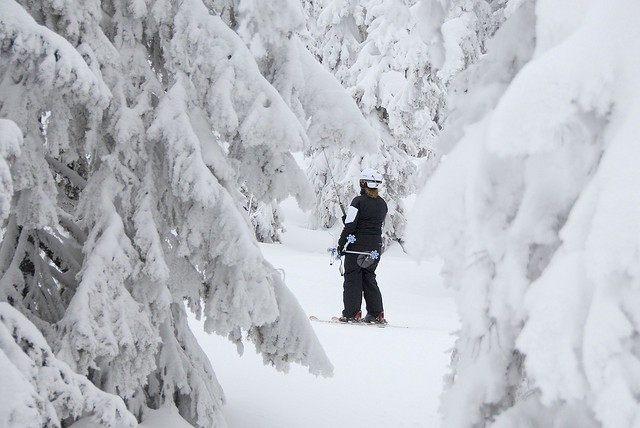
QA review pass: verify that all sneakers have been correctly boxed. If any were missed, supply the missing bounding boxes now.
[339,314,359,321]
[361,313,380,323]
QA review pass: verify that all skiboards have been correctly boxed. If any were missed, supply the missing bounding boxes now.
[309,316,408,329]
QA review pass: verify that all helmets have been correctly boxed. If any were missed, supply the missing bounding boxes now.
[359,168,383,189]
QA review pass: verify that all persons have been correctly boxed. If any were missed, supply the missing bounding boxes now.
[338,168,387,325]
